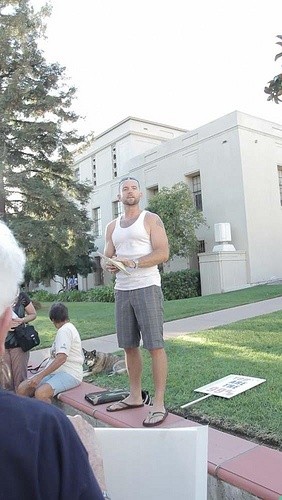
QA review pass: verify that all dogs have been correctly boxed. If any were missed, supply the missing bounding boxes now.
[82,348,128,377]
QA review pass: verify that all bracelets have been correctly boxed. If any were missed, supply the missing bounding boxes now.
[101,491,110,499]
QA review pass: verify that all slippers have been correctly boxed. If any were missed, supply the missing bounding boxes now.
[143,409,168,427]
[106,401,144,412]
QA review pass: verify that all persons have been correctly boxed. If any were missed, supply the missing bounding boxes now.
[2,291,36,395]
[100,177,169,426]
[18,303,83,404]
[0,221,110,500]
[66,276,79,291]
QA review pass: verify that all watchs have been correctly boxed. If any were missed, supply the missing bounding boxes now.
[131,258,139,269]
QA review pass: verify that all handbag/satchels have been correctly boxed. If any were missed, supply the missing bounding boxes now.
[13,325,40,352]
[85,389,130,406]
